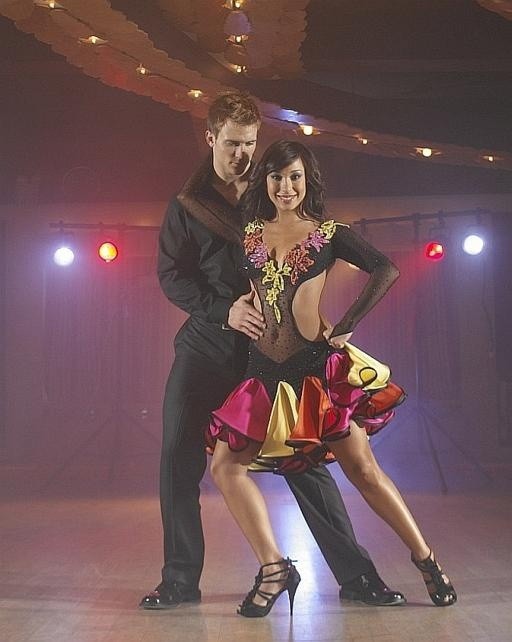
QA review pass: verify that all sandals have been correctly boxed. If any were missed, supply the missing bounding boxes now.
[411,542,458,607]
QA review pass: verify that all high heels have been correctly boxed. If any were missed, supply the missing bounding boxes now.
[237,556,301,618]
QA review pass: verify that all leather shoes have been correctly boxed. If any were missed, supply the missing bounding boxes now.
[139,577,201,609]
[339,570,408,607]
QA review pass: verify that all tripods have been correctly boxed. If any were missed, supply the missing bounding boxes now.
[47,221,209,498]
[352,206,493,494]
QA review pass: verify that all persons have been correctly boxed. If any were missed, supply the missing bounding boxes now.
[140,94,406,610]
[179,140,457,618]
